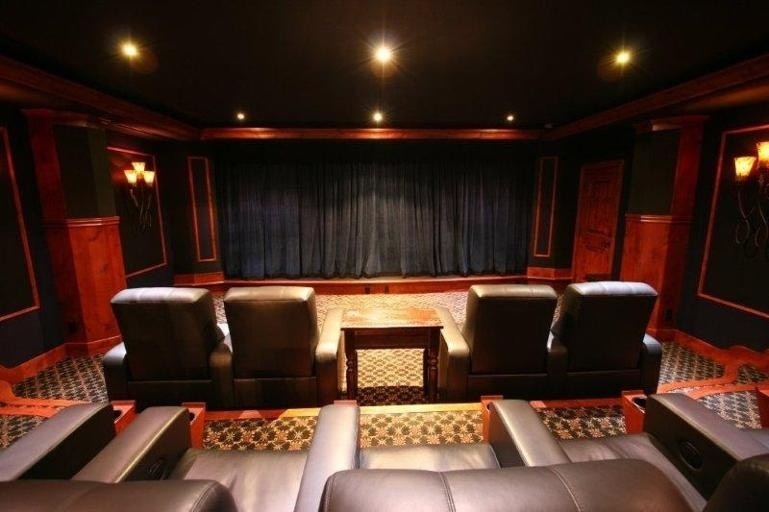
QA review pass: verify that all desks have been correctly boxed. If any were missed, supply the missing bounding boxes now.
[342,306,444,400]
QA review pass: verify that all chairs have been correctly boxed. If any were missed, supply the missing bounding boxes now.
[431,283,553,399]
[222,284,344,397]
[0,397,359,511]
[644,394,768,495]
[101,279,233,407]
[298,394,688,509]
[487,394,768,512]
[546,279,663,394]
[0,400,114,510]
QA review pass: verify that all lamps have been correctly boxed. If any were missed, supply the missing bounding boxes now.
[121,158,157,194]
